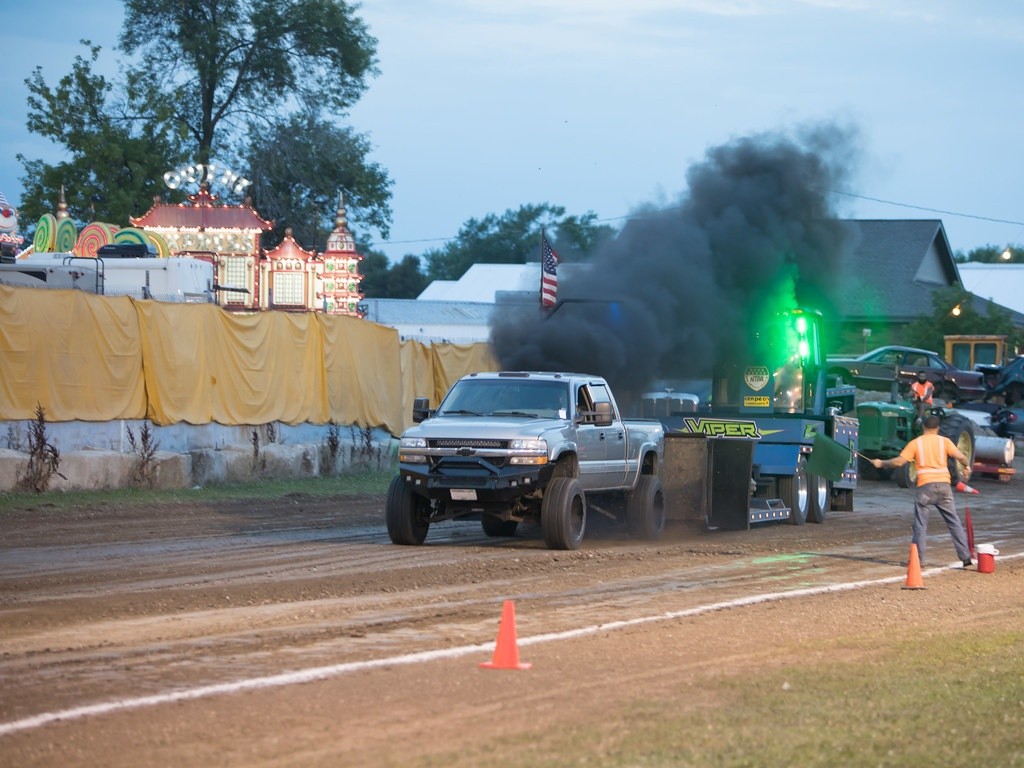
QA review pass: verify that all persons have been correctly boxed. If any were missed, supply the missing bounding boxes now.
[872,416,973,570]
[907,371,934,425]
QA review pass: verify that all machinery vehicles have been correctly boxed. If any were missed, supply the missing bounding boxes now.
[858,354,978,493]
[662,303,859,534]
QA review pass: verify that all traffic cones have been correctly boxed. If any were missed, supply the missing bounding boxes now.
[901,542,928,590]
[957,479,981,494]
[480,599,534,671]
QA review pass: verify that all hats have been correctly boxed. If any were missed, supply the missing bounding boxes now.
[925,416,939,428]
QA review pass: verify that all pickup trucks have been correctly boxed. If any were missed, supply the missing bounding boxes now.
[385,370,662,550]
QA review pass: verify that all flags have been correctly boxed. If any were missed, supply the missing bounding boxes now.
[806,432,850,482]
[540,236,560,308]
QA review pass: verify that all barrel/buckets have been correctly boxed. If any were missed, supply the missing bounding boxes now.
[974,544,1000,573]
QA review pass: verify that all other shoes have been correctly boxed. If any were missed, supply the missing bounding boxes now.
[916,419,921,424]
[963,558,972,567]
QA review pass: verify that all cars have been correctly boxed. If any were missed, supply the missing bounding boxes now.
[933,354,1024,434]
[827,344,987,410]
